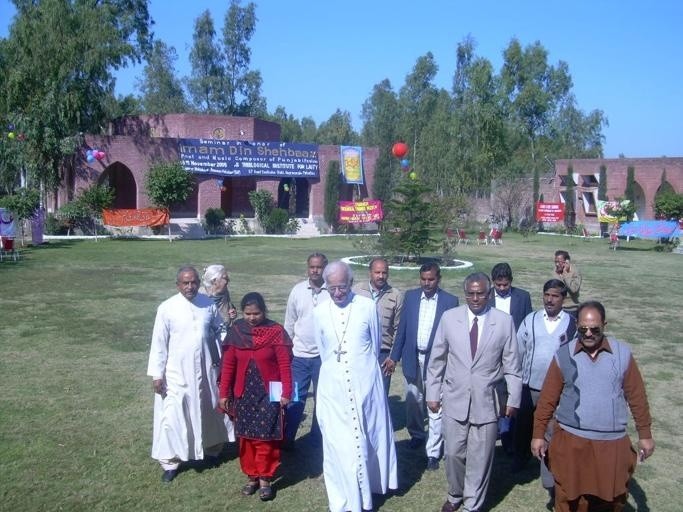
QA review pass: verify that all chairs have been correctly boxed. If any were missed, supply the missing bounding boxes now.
[0,236,17,263]
[445,226,503,248]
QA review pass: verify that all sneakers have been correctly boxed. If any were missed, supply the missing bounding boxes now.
[160,469,176,482]
[409,438,422,448]
[426,457,439,469]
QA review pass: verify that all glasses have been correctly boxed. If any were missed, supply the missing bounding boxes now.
[465,293,488,299]
[577,327,601,333]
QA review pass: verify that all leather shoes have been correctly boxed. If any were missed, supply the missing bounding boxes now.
[442,500,462,512]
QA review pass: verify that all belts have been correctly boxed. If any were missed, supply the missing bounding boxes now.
[418,347,429,355]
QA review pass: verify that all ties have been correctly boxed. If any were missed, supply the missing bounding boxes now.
[469,317,478,360]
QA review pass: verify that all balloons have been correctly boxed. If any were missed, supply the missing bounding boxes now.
[410,172,417,181]
[400,159,410,171]
[8,124,14,131]
[16,134,26,141]
[8,132,15,139]
[85,149,105,162]
[392,142,410,158]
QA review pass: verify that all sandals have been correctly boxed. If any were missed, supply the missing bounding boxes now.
[242,484,273,500]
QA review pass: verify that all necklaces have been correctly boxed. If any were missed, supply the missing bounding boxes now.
[329,300,352,362]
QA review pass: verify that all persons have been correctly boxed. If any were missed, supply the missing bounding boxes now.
[425,272,523,511]
[516,278,578,512]
[314,260,398,512]
[531,302,655,512]
[219,292,293,501]
[146,265,236,482]
[552,250,582,325]
[487,262,532,333]
[283,252,331,451]
[198,264,238,341]
[350,259,404,398]
[383,262,459,471]
